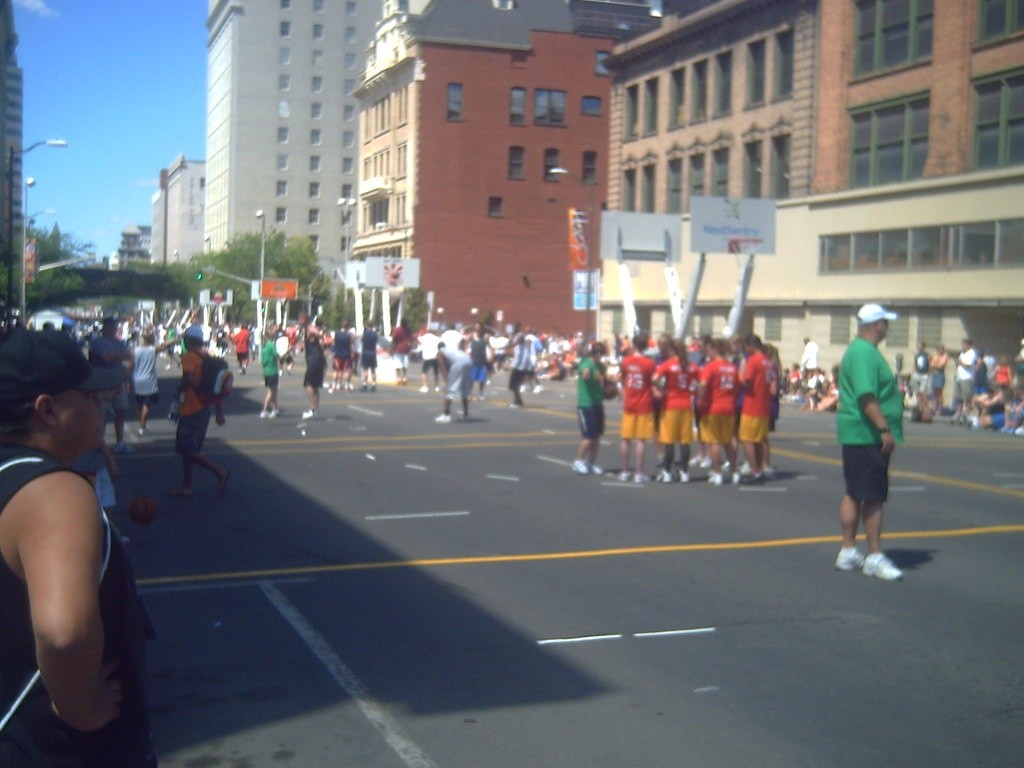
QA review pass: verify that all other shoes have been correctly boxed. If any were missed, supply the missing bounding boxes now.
[139,428,151,437]
[239,363,546,401]
[214,470,229,498]
[573,456,774,485]
[435,415,451,423]
[169,484,193,495]
[303,409,319,419]
[260,409,280,419]
[114,442,137,453]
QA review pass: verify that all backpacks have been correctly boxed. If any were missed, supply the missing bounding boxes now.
[195,351,235,405]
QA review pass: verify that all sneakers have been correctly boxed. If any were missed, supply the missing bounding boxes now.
[863,552,903,580]
[835,545,865,569]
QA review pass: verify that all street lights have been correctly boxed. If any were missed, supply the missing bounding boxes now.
[255,208,266,335]
[7,138,68,335]
[471,307,479,329]
[549,166,596,345]
[22,177,38,328]
[338,198,357,304]
[436,306,444,336]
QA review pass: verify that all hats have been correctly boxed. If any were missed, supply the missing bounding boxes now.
[0,327,126,407]
[859,304,897,326]
[182,327,205,339]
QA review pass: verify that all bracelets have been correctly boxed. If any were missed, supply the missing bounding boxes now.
[879,426,890,433]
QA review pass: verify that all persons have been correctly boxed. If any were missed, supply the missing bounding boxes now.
[42,315,190,451]
[898,340,948,422]
[0,330,161,767]
[418,322,584,422]
[951,338,1024,436]
[203,313,328,420]
[169,327,234,496]
[836,304,904,580]
[782,338,839,412]
[572,332,780,485]
[323,321,414,392]
